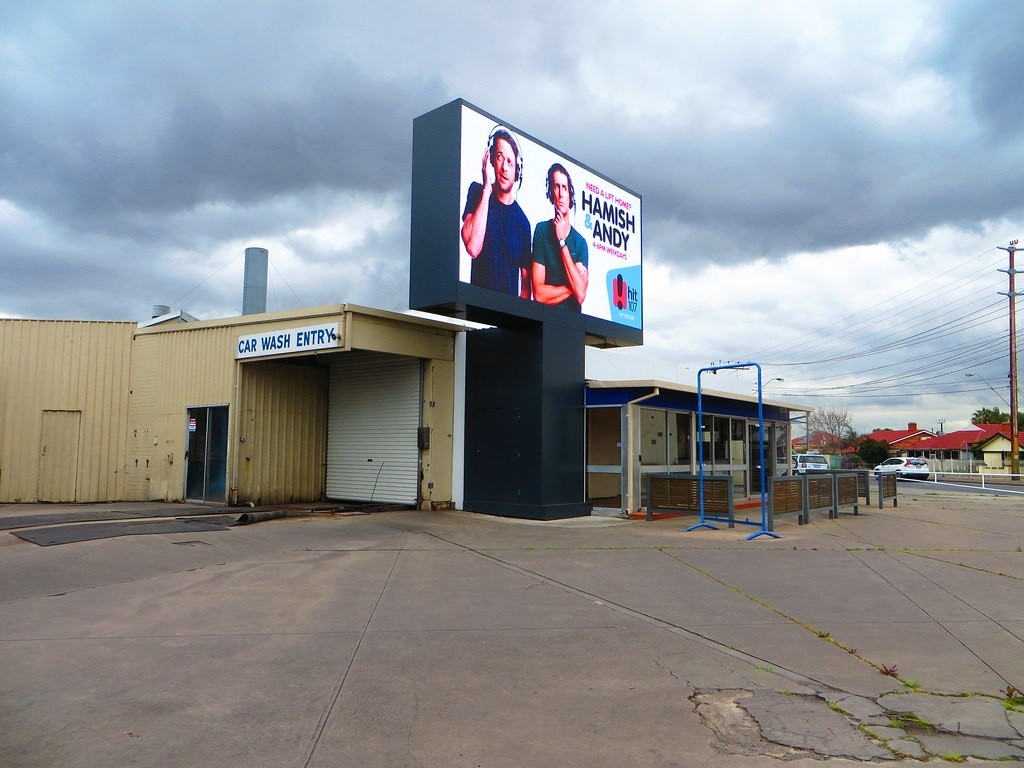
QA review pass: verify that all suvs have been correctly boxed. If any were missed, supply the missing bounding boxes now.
[790,454,829,476]
[873,457,929,478]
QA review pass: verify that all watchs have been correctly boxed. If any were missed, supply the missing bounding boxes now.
[559,238,568,248]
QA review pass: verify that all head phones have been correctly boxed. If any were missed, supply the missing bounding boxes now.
[544,164,576,208]
[488,123,523,182]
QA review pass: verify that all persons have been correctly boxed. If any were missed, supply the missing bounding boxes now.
[461,130,532,301]
[531,163,588,313]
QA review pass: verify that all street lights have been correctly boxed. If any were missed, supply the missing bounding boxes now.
[965,372,1021,481]
